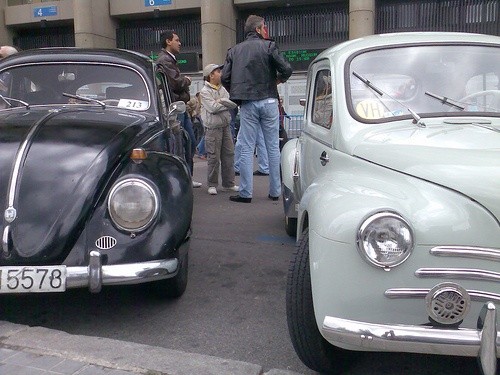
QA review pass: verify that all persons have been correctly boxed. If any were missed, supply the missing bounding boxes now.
[187,91,238,159]
[199,62,241,195]
[0,46,19,110]
[155,31,203,187]
[221,14,293,203]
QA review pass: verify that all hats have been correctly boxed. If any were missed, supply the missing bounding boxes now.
[202,64,223,77]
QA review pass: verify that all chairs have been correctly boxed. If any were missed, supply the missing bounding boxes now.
[21,90,55,105]
[105,86,137,105]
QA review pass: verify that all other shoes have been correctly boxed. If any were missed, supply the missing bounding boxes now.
[192,181,202,187]
[220,185,239,192]
[234,172,241,176]
[196,147,205,160]
[229,195,252,203]
[208,186,218,195]
[253,169,270,175]
[268,194,279,201]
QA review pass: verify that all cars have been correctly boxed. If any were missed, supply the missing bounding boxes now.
[0,44,195,301]
[278,30,500,375]
